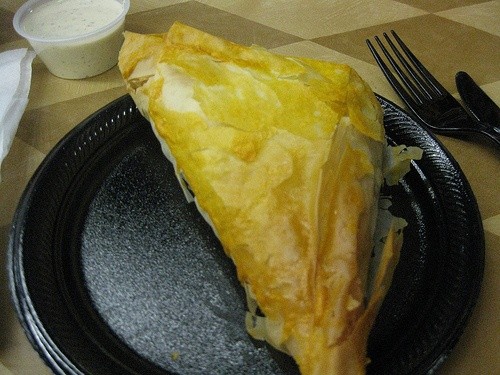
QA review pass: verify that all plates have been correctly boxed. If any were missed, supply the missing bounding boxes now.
[6,93,487,375]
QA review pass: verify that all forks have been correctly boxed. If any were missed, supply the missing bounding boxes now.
[364,28,500,152]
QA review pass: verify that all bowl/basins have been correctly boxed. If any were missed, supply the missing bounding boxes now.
[12,0,130,80]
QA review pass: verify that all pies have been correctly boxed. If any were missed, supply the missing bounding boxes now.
[119,21,424,375]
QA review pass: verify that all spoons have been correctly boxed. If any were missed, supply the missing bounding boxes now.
[455,70,500,133]
